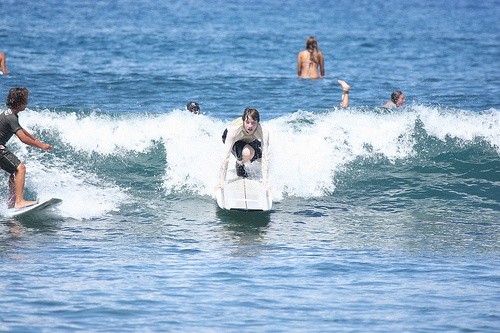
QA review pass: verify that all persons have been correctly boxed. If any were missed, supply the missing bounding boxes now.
[296,36,325,79]
[0,50,352,113]
[383,91,404,112]
[215,107,270,190]
[0,88,54,211]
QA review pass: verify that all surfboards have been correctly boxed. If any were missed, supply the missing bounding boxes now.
[0,197,63,219]
[214,177,273,212]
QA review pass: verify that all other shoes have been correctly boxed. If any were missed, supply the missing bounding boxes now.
[236,162,248,178]
